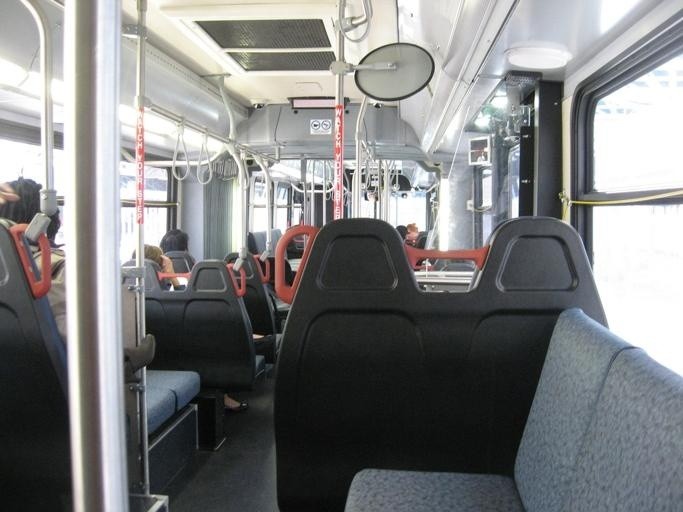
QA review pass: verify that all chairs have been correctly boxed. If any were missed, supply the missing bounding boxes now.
[0,219,267,510]
[268,215,683,512]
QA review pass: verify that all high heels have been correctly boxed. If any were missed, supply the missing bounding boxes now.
[124,334,156,382]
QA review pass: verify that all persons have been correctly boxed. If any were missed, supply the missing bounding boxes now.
[407,224,418,243]
[132,244,273,412]
[160,230,197,265]
[396,226,415,248]
[477,152,486,162]
[0,179,156,386]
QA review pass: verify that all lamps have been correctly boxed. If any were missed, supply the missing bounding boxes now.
[474,45,573,129]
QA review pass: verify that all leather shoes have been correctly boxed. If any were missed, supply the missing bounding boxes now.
[223,401,248,412]
